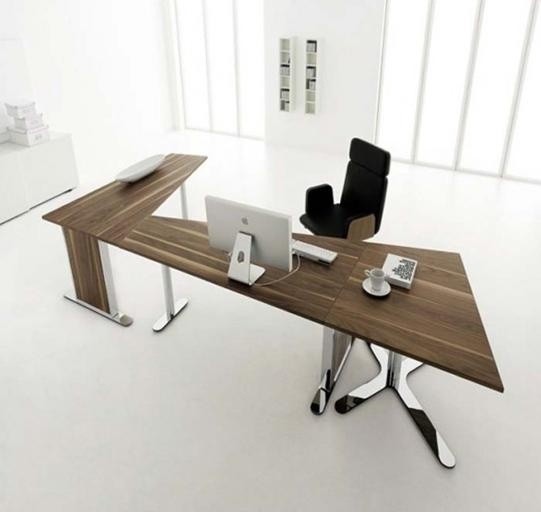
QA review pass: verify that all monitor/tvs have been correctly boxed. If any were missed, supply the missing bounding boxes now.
[204,195,293,288]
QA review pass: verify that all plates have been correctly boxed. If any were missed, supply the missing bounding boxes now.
[361,278,390,297]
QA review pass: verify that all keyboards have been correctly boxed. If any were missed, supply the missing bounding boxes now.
[292,239,339,267]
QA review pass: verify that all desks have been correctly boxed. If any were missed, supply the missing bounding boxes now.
[119,215,509,469]
[40,153,209,328]
[1,117,78,226]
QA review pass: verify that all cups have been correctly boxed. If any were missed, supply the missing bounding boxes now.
[364,268,385,288]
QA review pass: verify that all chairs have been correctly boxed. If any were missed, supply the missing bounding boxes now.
[299,139,390,240]
